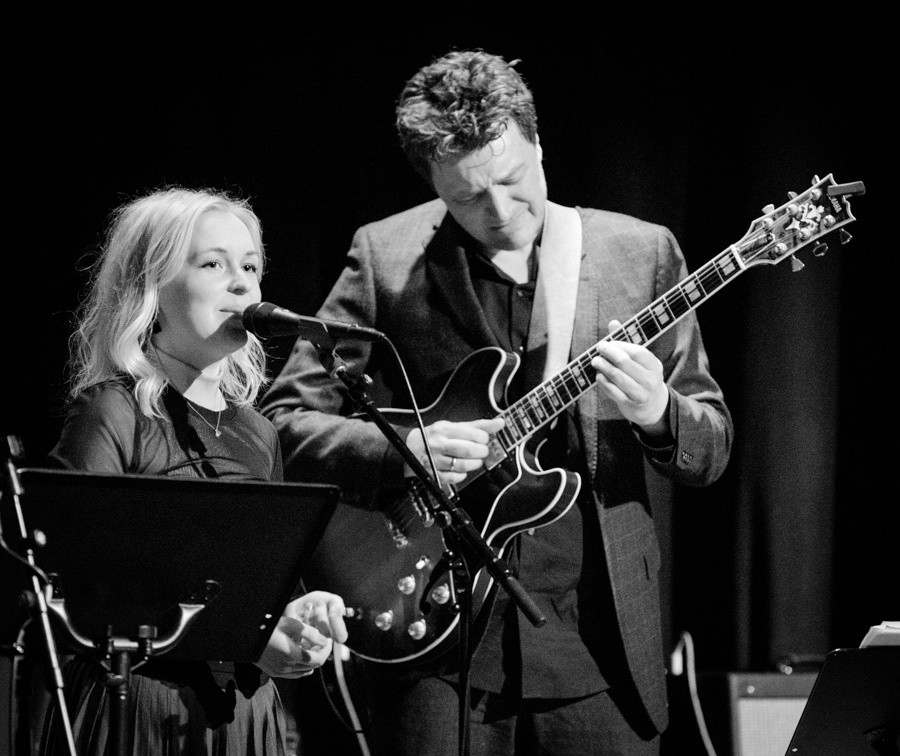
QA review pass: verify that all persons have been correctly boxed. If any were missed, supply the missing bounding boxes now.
[22,189,350,756]
[262,55,732,756]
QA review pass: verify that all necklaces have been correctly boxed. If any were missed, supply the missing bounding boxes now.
[149,340,224,437]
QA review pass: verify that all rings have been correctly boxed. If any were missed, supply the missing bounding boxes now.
[450,457,456,472]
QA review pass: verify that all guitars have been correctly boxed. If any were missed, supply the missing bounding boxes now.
[295,164,869,674]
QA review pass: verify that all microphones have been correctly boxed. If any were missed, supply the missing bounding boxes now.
[242,301,384,342]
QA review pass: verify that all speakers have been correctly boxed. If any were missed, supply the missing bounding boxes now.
[708,667,821,756]
